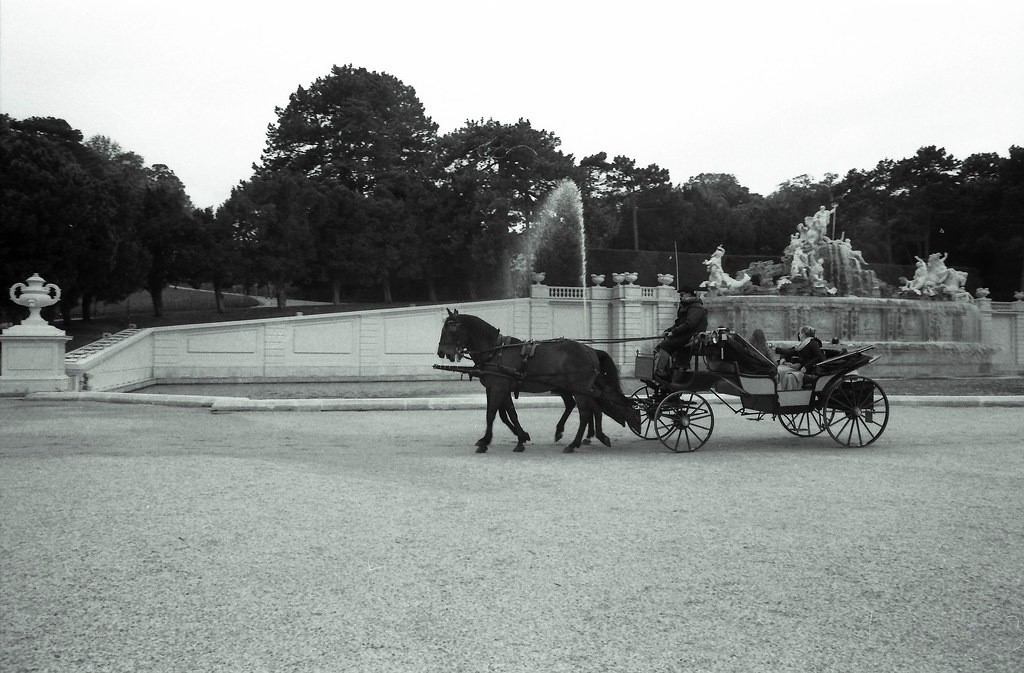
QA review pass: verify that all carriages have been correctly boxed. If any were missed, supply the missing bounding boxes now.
[431,307,891,456]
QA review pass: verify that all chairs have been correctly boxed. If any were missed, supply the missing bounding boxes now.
[672,333,726,373]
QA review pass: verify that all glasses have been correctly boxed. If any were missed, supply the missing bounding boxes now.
[680,293,686,297]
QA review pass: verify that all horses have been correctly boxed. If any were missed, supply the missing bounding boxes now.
[436,308,640,454]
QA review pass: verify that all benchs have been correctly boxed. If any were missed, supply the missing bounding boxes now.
[801,348,848,388]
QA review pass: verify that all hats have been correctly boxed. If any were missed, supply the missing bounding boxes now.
[677,284,696,296]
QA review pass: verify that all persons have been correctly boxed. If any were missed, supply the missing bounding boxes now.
[702,246,725,289]
[789,205,868,283]
[748,326,826,392]
[898,253,968,297]
[653,286,708,382]
